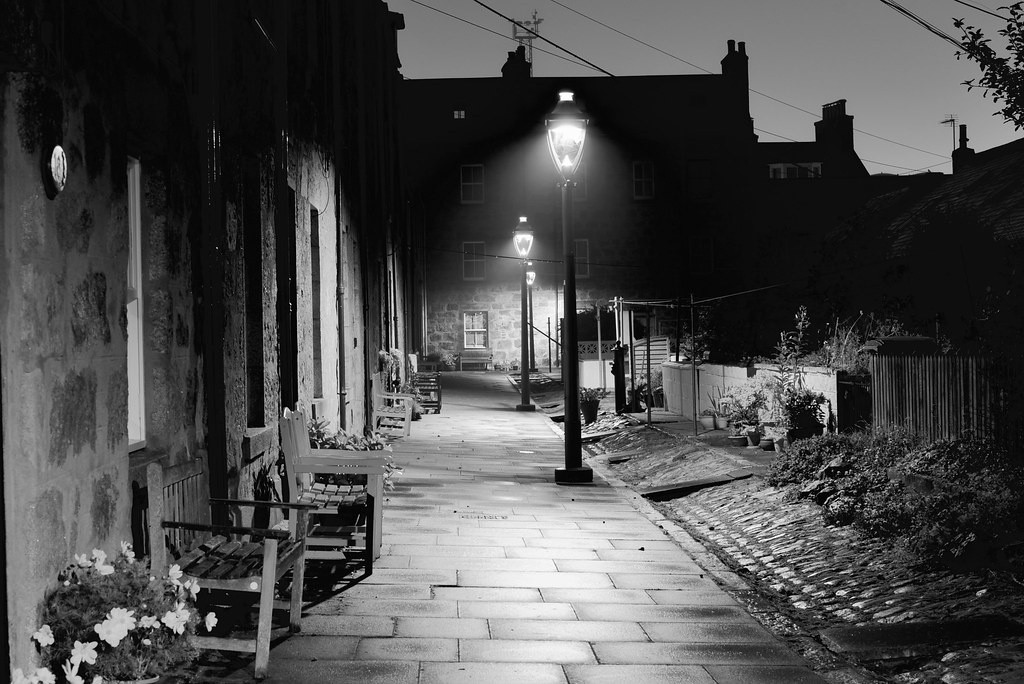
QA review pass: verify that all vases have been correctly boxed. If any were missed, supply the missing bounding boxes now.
[102,672,161,684]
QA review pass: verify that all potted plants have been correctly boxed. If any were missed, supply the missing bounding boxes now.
[698,386,730,430]
[720,368,829,452]
[579,386,612,425]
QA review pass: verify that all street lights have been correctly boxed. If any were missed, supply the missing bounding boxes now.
[513,217,538,412]
[525,261,541,373]
[543,90,599,485]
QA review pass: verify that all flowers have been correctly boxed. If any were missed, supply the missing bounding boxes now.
[11,538,218,684]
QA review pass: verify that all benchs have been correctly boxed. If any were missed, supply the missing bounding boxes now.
[280,407,394,562]
[372,372,416,436]
[405,352,443,414]
[147,449,319,678]
[458,350,492,372]
